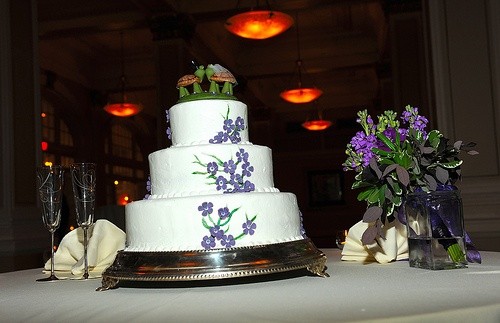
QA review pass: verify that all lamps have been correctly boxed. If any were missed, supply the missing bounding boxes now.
[280,9,322,103]
[301,100,331,131]
[223,0,295,40]
[101,31,143,118]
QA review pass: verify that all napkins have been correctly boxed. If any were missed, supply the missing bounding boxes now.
[44,219,127,279]
[340,215,423,262]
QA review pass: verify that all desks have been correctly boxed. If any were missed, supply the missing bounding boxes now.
[0,249,500,323]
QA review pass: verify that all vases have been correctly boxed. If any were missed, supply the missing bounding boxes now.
[403,190,467,270]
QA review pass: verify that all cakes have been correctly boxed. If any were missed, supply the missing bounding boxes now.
[124,63,305,252]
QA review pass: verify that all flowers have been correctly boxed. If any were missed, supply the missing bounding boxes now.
[343,105,483,263]
